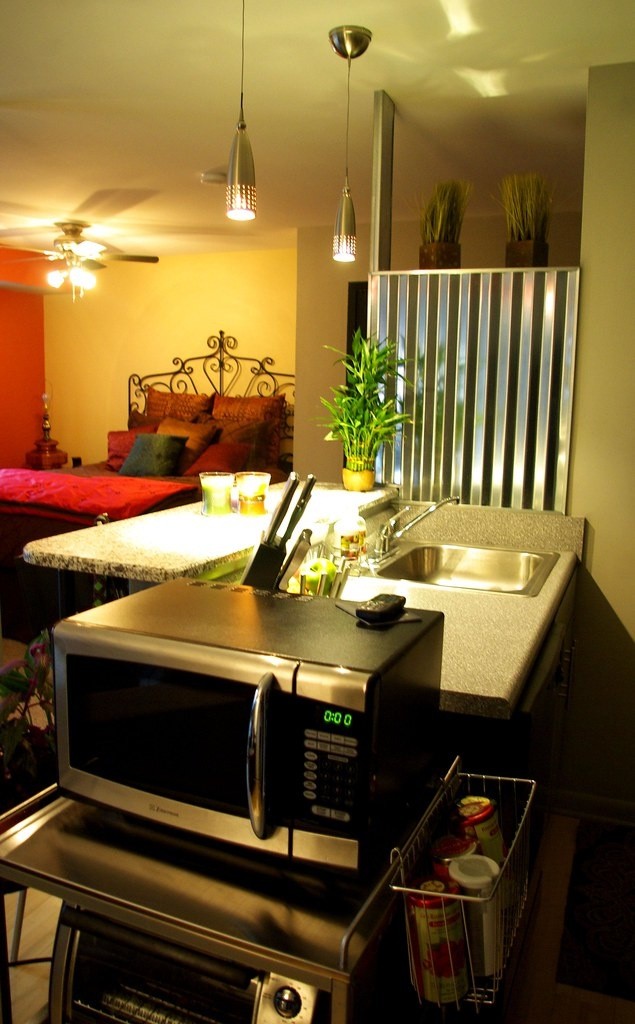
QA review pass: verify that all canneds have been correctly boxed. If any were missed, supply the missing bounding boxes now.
[406,797,518,1003]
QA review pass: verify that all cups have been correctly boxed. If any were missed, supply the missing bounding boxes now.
[199,473,236,514]
[236,472,271,515]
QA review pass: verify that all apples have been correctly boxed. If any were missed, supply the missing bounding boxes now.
[287,557,338,598]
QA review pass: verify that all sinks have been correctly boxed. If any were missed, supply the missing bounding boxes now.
[378,544,544,592]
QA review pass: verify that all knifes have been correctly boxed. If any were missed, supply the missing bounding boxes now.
[261,473,316,593]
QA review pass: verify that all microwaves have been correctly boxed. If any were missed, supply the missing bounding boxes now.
[52,580,445,893]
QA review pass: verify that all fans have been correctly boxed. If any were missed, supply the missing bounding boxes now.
[0,223,159,301]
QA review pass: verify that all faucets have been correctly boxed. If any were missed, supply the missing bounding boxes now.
[375,496,460,552]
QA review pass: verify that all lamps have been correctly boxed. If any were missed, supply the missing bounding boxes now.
[329,25,372,262]
[46,258,95,290]
[223,0,257,221]
[42,394,51,441]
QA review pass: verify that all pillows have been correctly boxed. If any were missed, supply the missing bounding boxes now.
[106,384,286,477]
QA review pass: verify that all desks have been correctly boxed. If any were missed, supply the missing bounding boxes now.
[27,450,67,470]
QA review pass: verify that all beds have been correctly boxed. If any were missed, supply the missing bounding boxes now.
[0,329,295,645]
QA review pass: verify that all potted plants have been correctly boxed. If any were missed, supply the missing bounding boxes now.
[492,170,552,267]
[403,179,472,269]
[308,326,418,492]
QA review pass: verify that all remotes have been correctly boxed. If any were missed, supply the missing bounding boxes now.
[354,593,407,622]
